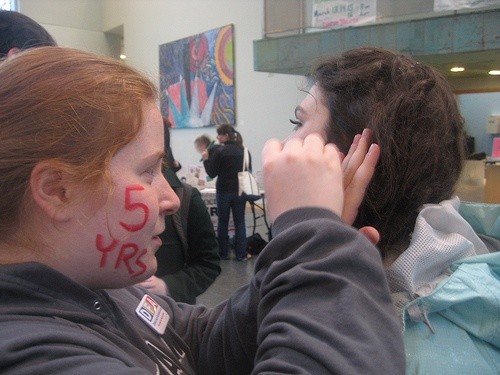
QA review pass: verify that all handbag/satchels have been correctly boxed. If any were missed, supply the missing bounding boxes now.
[237,171,261,201]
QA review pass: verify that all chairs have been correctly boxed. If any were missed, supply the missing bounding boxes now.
[238,171,271,235]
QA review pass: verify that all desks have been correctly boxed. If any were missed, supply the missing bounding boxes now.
[200,188,235,232]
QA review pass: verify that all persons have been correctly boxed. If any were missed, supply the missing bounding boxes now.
[0,48,405,375]
[138,122,222,306]
[195,124,253,263]
[288,48,500,375]
[0,10,57,60]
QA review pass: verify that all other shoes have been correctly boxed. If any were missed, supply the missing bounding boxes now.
[237,254,251,261]
